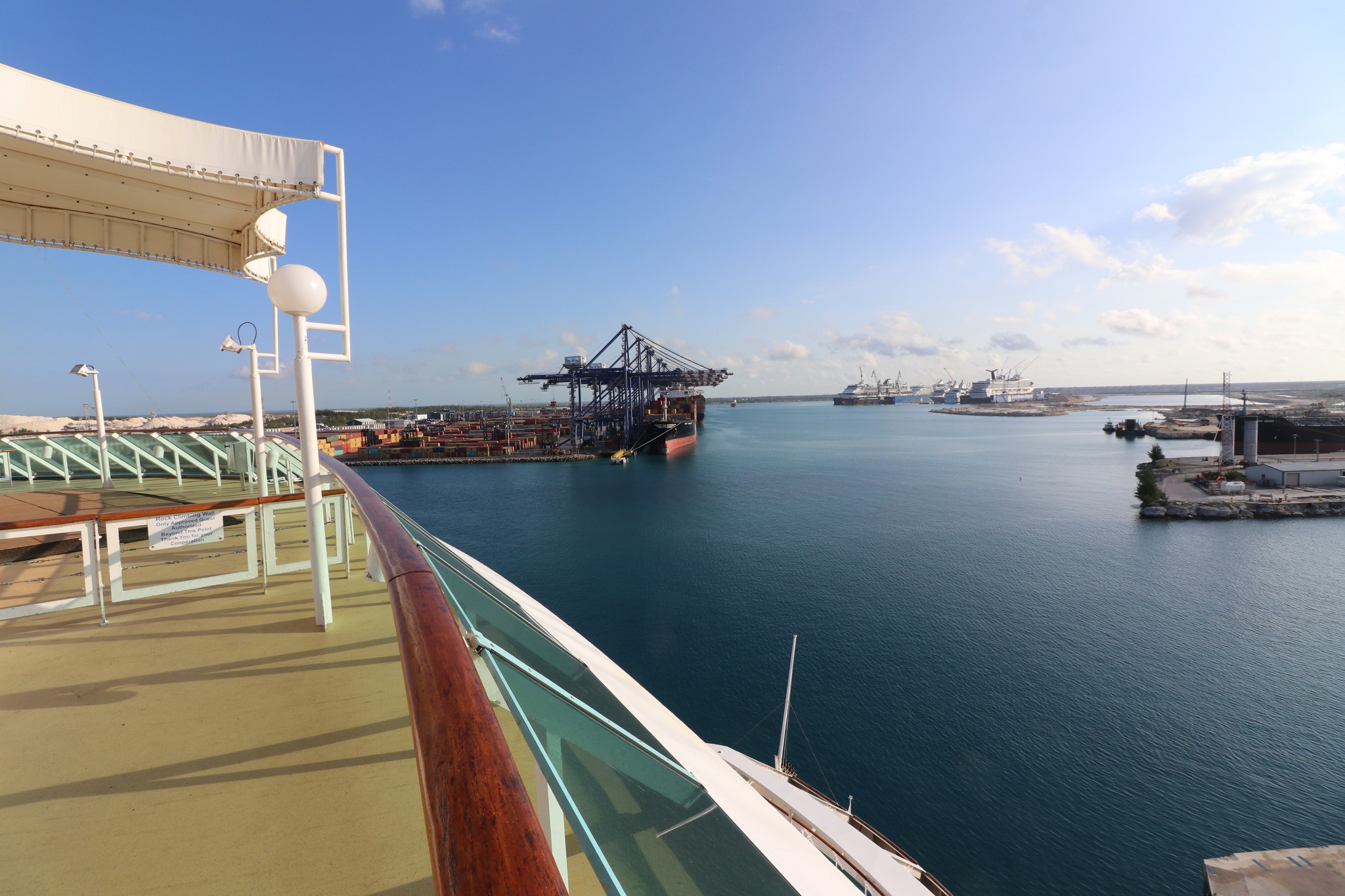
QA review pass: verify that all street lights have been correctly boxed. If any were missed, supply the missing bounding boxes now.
[490,401,492,411]
[462,400,464,422]
[413,399,418,428]
[290,400,297,438]
[534,400,536,411]
[480,401,484,417]
[519,400,522,420]
[69,364,114,489]
[531,399,533,417]
[515,400,517,415]
[459,402,460,414]
[220,334,268,499]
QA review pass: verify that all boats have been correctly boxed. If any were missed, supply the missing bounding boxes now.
[730,399,737,407]
[657,382,706,425]
[1214,389,1345,456]
[1102,417,1115,433]
[640,391,697,455]
[832,369,1035,405]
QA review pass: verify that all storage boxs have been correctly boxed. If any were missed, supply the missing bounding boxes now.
[286,395,706,462]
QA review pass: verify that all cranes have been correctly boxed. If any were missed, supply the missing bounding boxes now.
[516,322,735,452]
[500,377,515,416]
